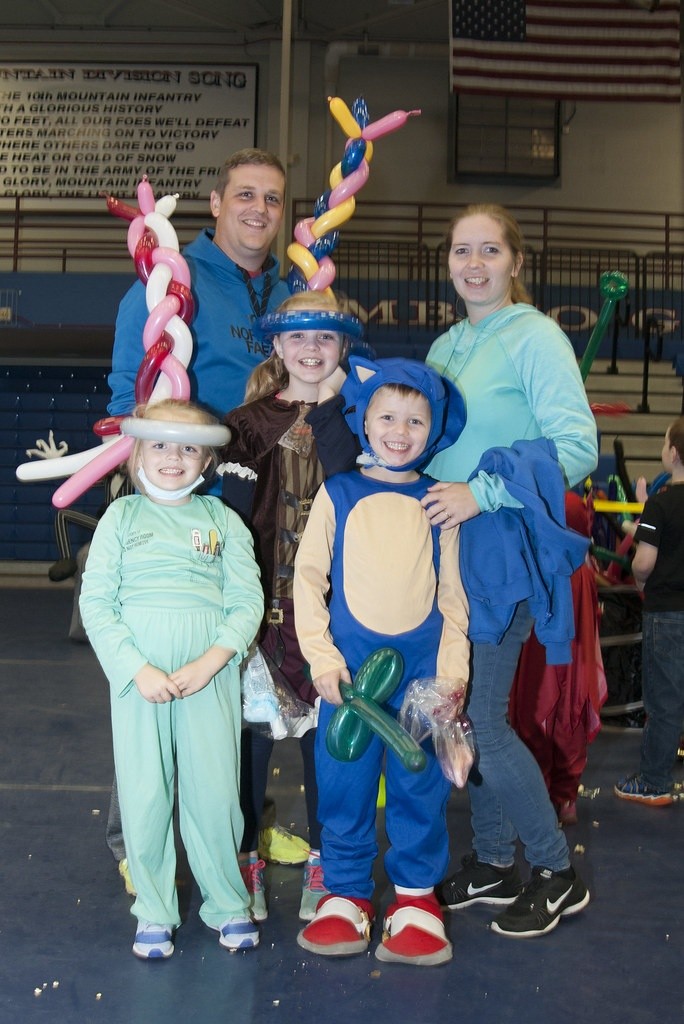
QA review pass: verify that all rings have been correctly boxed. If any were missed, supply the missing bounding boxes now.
[443,510,450,518]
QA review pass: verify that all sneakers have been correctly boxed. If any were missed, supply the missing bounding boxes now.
[614,775,674,806]
[434,850,518,911]
[133,917,175,959]
[240,856,269,923]
[491,866,592,937]
[220,920,261,949]
[258,823,311,866]
[298,849,332,921]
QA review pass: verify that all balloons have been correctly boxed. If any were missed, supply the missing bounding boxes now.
[325,648,427,773]
[17,173,230,508]
[583,436,672,588]
[580,272,629,384]
[252,97,421,352]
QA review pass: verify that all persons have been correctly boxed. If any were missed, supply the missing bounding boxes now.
[224,290,364,918]
[428,207,595,937]
[292,351,469,969]
[612,415,684,802]
[80,396,267,957]
[108,147,295,881]
